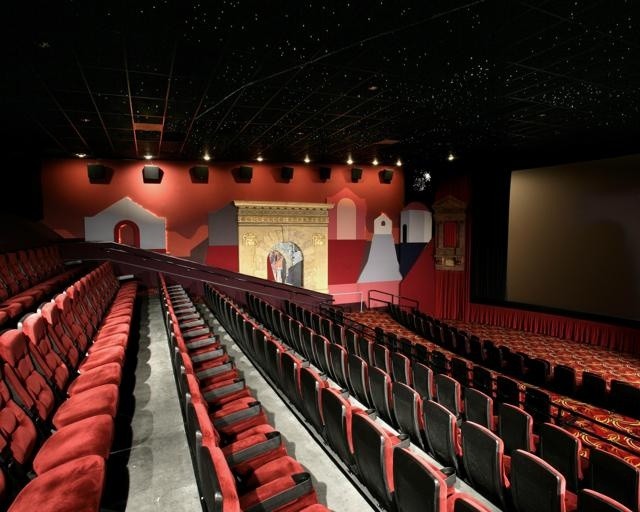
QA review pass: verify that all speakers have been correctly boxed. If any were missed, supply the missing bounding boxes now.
[383,169,392,181]
[239,165,252,178]
[87,162,107,182]
[190,165,208,183]
[144,165,160,182]
[352,167,362,179]
[320,167,331,179]
[282,168,293,179]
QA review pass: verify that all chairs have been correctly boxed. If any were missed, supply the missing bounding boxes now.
[0,245,639,512]
